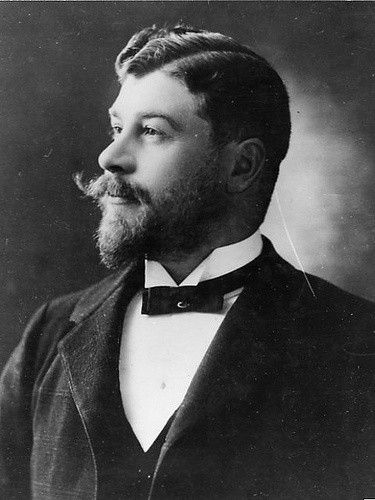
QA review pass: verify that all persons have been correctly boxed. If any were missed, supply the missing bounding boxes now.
[0,24,375,500]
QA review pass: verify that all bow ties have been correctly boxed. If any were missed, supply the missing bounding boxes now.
[141,286,225,316]
